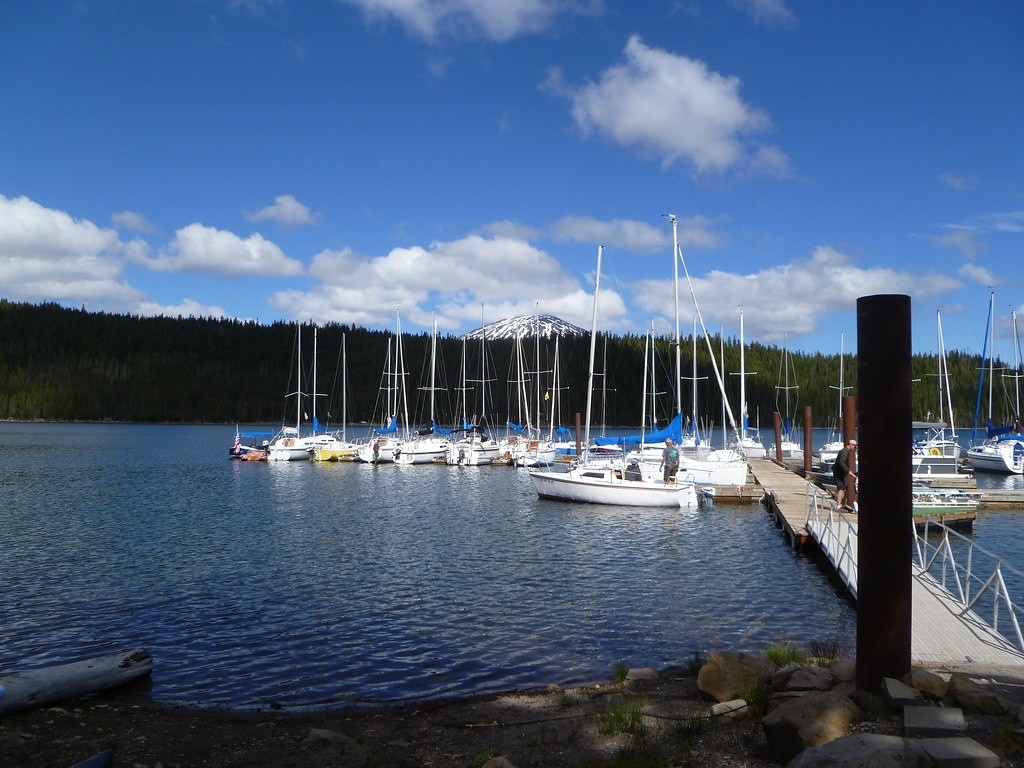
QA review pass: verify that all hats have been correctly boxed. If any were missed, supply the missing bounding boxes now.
[849,439,858,448]
[631,458,638,465]
[665,438,672,442]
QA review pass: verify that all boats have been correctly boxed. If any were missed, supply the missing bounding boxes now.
[228,425,264,457]
[911,421,960,476]
[912,490,984,527]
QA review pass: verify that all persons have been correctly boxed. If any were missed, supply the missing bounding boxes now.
[1014,419,1022,436]
[830,439,858,513]
[660,437,680,482]
[624,460,642,481]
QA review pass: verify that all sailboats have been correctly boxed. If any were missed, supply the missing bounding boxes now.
[815,332,852,463]
[911,309,963,460]
[527,244,698,507]
[555,306,798,462]
[631,214,748,486]
[264,309,362,462]
[500,300,583,468]
[967,290,1024,474]
[357,303,503,467]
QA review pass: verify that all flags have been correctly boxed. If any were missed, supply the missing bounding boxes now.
[233,435,241,454]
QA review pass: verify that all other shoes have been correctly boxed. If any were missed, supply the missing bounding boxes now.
[836,507,848,512]
